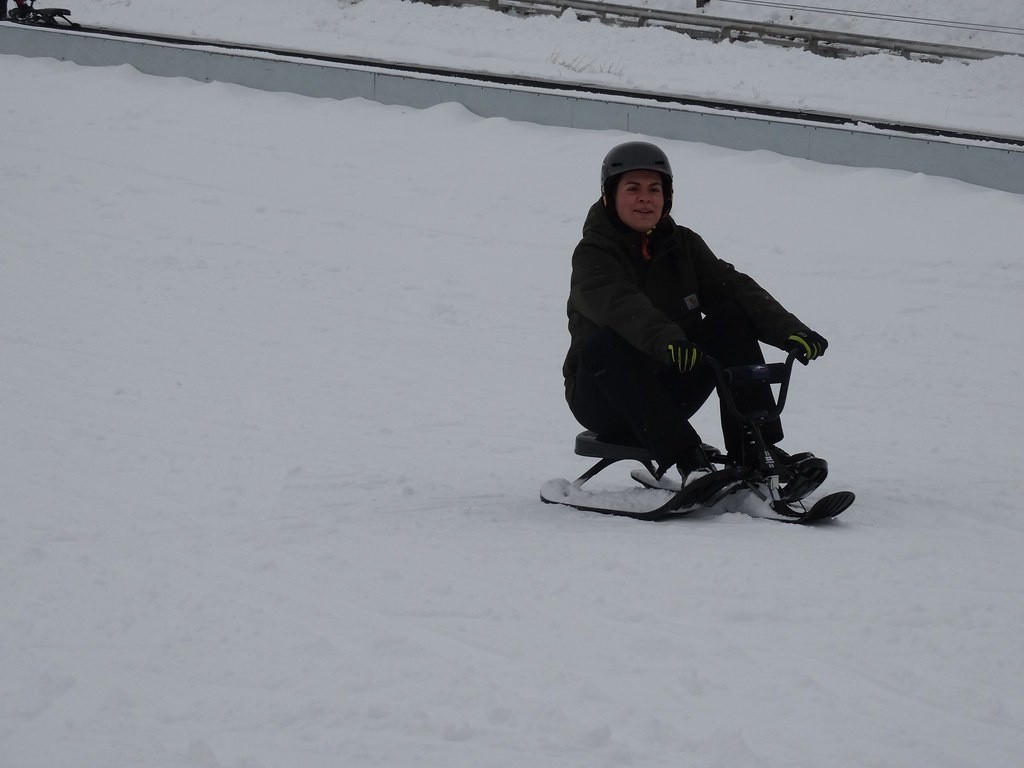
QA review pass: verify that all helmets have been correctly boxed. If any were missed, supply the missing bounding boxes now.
[601,141,673,209]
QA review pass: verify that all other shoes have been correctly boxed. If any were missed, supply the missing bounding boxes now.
[728,448,814,479]
[673,446,718,489]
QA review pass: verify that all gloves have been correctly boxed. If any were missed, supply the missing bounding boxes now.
[667,342,701,374]
[787,326,827,365]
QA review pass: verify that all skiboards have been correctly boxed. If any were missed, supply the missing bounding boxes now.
[534,462,859,527]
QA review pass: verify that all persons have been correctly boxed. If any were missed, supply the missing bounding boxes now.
[563,141,827,509]
